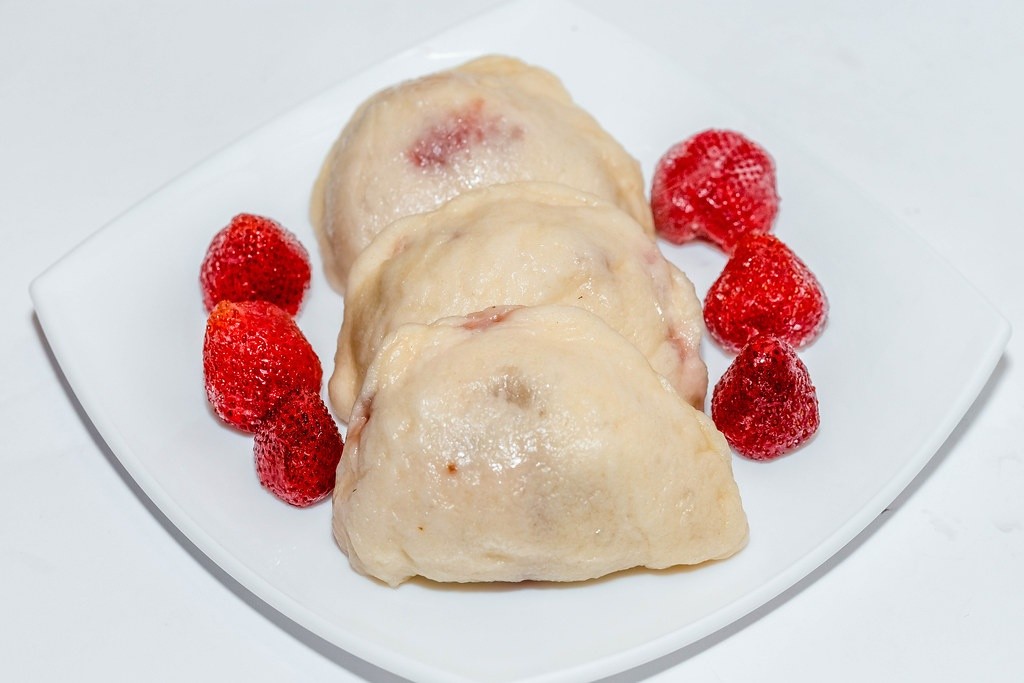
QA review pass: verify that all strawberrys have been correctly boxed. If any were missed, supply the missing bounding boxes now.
[650,128,830,462]
[198,212,346,506]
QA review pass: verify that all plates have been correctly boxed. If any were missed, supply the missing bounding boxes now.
[31,1,1011,683]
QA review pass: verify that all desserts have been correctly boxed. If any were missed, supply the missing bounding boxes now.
[306,51,752,586]
